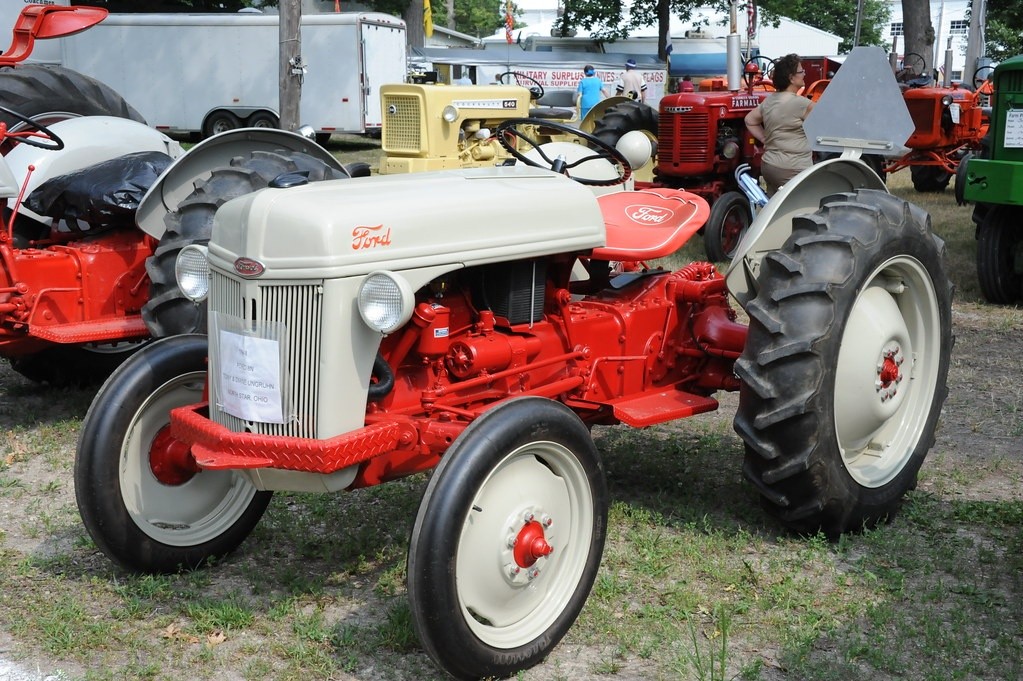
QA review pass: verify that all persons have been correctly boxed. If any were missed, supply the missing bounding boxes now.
[616,60,647,104]
[458,73,472,85]
[495,74,502,85]
[576,64,611,121]
[670,75,694,92]
[744,55,815,200]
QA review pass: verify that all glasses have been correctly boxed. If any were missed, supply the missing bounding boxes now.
[790,70,806,75]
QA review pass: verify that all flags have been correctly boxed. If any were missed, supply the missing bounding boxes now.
[506,0,512,44]
[424,0,433,38]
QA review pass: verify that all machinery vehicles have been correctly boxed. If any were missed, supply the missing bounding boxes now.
[0,3,187,387]
[648,0,993,263]
[962,54,1023,304]
[344,71,659,179]
[73,117,955,681]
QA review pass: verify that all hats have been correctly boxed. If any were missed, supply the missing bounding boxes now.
[584,65,595,75]
[624,59,636,69]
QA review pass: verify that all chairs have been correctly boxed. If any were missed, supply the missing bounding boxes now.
[528,88,582,122]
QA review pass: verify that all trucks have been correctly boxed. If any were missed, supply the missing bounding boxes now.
[0,1,407,147]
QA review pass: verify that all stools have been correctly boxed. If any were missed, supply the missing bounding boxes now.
[591,188,710,262]
